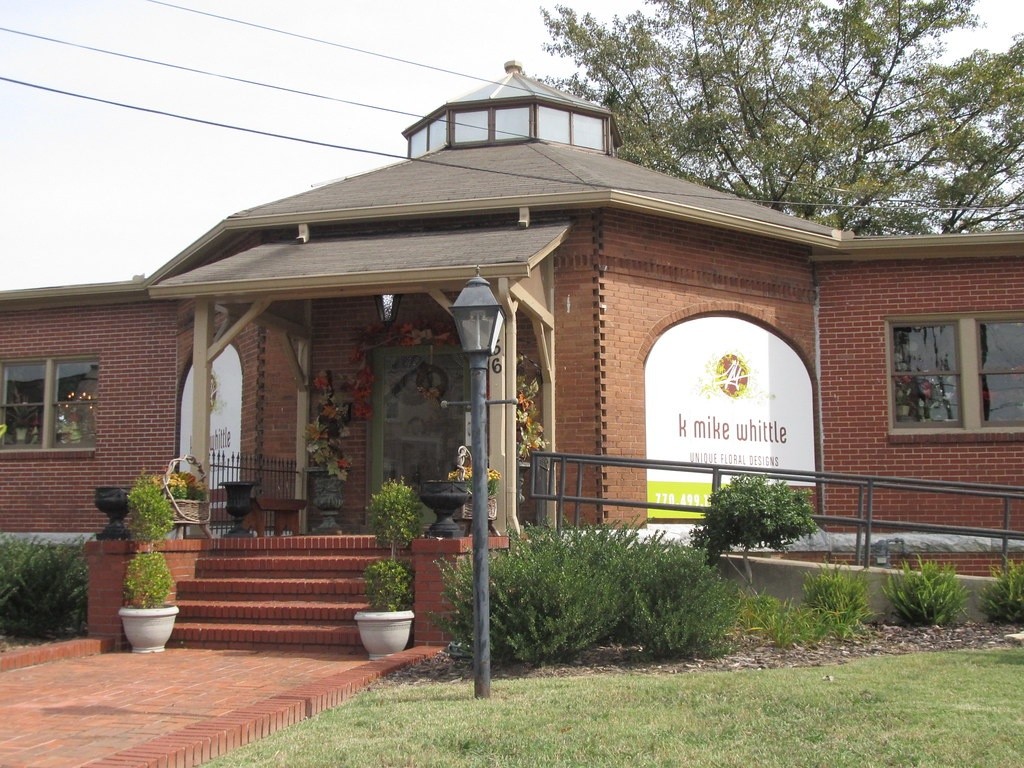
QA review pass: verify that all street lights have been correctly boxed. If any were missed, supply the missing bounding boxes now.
[445,264,508,701]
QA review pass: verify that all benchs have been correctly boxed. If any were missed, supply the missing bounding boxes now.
[244,498,307,537]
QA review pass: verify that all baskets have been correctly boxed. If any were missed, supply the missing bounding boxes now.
[453,445,497,521]
[161,454,210,525]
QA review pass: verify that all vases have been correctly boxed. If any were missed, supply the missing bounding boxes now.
[307,467,353,535]
[14,426,28,445]
[453,446,501,537]
[516,461,531,507]
[896,406,909,416]
[163,454,213,540]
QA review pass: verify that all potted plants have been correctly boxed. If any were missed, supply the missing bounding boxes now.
[118,465,180,654]
[353,476,426,661]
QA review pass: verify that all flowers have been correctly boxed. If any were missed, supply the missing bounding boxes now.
[168,472,207,501]
[301,320,550,484]
[448,467,502,498]
[895,376,911,405]
